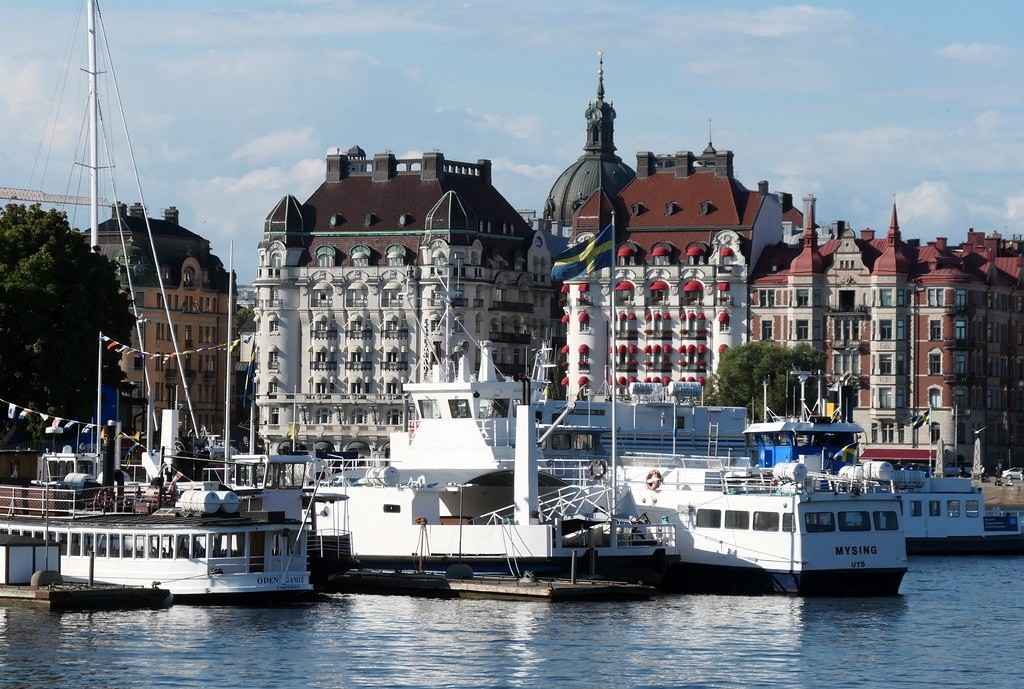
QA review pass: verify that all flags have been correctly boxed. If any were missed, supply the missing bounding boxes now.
[910,409,930,428]
[550,221,612,282]
[8,333,256,493]
[833,442,858,460]
[543,387,548,401]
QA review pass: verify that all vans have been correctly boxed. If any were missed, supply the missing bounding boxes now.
[944,461,985,476]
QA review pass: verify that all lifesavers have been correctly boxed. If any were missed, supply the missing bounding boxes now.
[96,487,115,509]
[588,459,608,481]
[156,487,177,507]
[644,469,663,491]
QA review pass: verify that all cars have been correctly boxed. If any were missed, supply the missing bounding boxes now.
[1002,467,1024,480]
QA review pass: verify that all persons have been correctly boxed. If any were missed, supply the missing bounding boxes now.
[960,462,1024,487]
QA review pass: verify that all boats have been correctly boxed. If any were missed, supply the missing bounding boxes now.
[0,2,1024,607]
